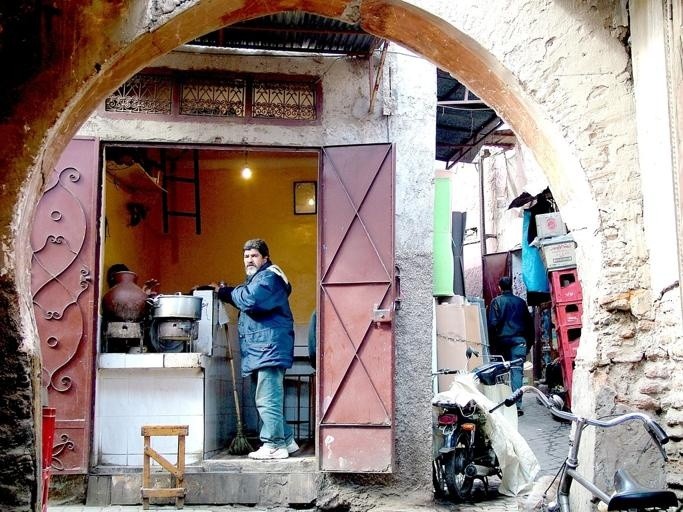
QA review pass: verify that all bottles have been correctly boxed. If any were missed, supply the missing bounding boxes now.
[218,280,227,288]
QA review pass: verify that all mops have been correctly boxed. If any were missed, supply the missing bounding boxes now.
[218,282,256,454]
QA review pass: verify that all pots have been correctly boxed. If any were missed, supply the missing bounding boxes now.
[145,292,202,319]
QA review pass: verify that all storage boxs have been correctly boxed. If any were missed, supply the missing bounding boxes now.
[535,212,578,273]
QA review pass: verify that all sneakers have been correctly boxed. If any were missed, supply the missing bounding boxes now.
[249,439,299,460]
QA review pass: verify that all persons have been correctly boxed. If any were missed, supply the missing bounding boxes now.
[208,239,300,459]
[487,276,535,417]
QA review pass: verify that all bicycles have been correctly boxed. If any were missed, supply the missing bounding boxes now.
[488,385,683,512]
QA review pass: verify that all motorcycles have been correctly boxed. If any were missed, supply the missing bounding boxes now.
[432,346,523,505]
